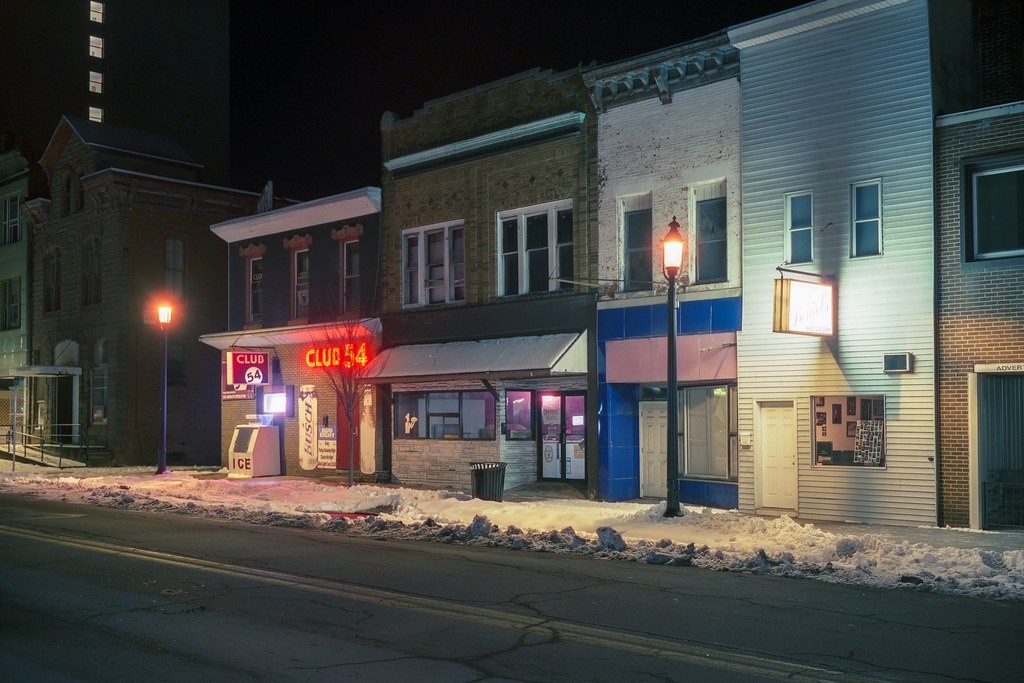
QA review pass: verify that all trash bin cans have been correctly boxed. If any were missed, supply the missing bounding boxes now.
[468,462,508,503]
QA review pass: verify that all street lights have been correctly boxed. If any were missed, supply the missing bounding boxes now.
[155,301,168,475]
[656,214,686,520]
